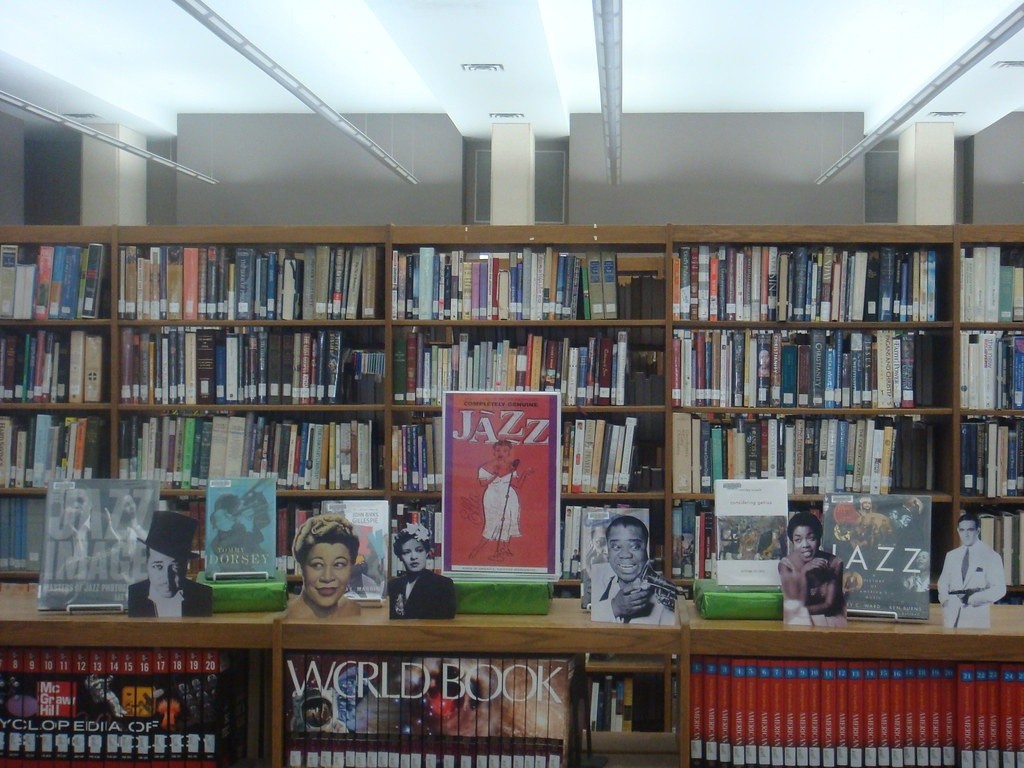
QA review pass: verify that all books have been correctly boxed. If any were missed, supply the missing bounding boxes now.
[284,655,576,768]
[820,493,931,619]
[712,478,789,585]
[0,645,246,768]
[589,673,633,732]
[440,390,562,583]
[204,477,276,582]
[690,654,1024,768]
[580,508,650,609]
[0,242,666,579]
[671,244,1024,587]
[321,500,389,607]
[36,479,160,612]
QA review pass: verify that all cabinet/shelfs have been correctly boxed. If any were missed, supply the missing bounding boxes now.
[1,223,1024,768]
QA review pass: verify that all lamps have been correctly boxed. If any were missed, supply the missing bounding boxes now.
[1,89,221,186]
[174,0,422,186]
[814,2,1024,188]
[591,0,623,189]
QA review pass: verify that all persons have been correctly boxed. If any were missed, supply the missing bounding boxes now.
[591,516,676,626]
[777,512,848,627]
[387,523,456,619]
[580,511,621,608]
[287,513,361,619]
[936,513,1006,628]
[128,511,213,617]
